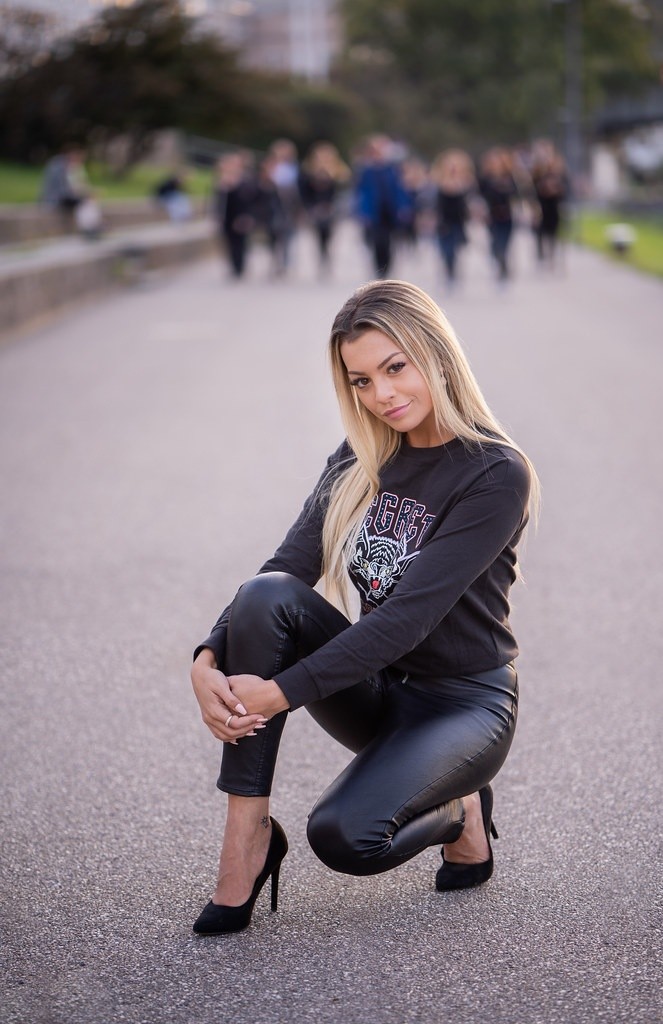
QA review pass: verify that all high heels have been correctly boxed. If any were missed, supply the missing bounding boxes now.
[436,783,498,892]
[193,816,289,935]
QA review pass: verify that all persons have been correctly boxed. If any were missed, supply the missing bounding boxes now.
[39,120,571,283]
[190,277,546,936]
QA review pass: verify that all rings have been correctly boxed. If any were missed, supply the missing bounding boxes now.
[224,714,235,727]
